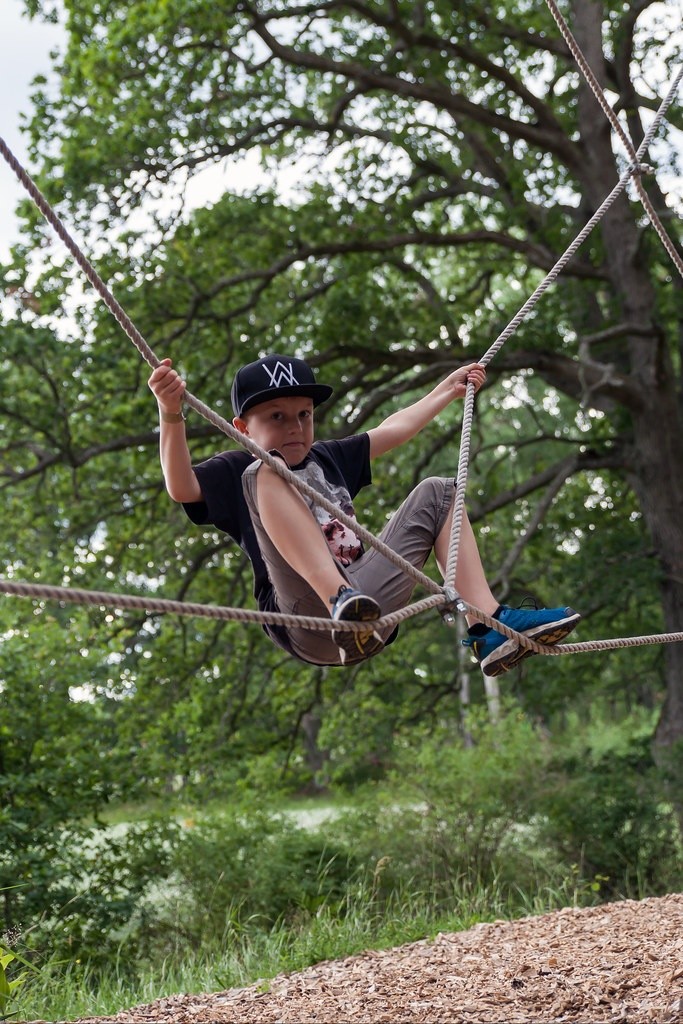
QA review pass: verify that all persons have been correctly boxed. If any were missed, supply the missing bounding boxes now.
[146,356,581,678]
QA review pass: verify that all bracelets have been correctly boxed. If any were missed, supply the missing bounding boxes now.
[158,411,187,424]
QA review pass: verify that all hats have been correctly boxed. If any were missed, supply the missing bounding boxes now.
[229,353,333,419]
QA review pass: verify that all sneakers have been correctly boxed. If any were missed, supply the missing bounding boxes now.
[459,596,582,676]
[329,584,381,667]
